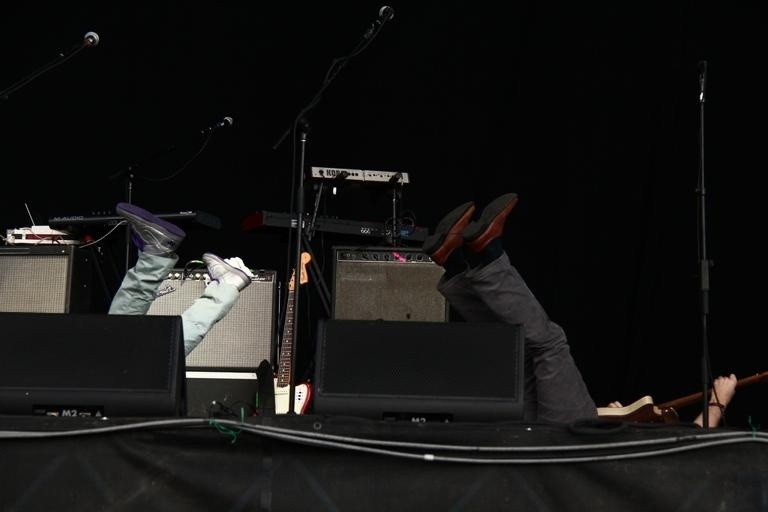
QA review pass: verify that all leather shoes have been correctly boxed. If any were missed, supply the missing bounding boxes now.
[462,191,520,254]
[422,200,475,267]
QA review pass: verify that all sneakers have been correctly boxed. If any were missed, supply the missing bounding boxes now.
[116,202,186,258]
[202,252,252,292]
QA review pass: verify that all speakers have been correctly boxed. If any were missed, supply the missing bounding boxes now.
[334,245,452,319]
[315,318,526,420]
[0,247,76,308]
[0,310,189,418]
[134,267,278,373]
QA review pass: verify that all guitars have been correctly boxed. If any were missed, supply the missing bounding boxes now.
[597,372,768,423]
[273,251,311,415]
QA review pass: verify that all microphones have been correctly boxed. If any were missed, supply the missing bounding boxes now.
[68,30,99,55]
[378,7,396,23]
[201,116,235,136]
[697,60,709,103]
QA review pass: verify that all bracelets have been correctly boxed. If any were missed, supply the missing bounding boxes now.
[707,402,726,411]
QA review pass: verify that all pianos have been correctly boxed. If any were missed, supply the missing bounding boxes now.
[237,208,427,244]
[49,210,221,231]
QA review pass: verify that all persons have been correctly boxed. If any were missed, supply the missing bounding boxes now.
[422,194,738,428]
[107,201,249,357]
